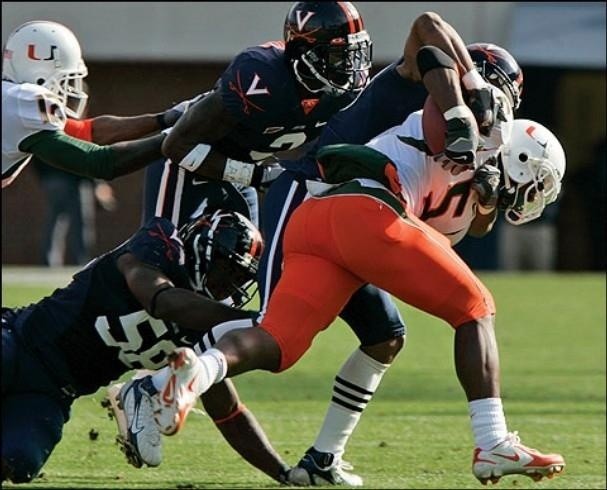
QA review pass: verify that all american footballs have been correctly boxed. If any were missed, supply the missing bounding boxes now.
[423,90,474,173]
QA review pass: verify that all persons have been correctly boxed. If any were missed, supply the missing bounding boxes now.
[0,212,291,489]
[26,154,119,267]
[99,9,523,486]
[1,18,211,193]
[139,0,372,292]
[153,46,562,485]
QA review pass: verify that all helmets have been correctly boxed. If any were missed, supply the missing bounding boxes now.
[499,119,566,223]
[177,210,264,311]
[284,0,372,96]
[467,42,524,108]
[0,21,89,118]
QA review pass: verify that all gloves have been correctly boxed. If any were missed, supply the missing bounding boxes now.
[256,148,302,192]
[163,90,213,128]
[432,105,480,174]
[470,163,502,202]
[463,69,508,127]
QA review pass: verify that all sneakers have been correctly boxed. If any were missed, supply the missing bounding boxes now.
[153,346,203,437]
[288,457,363,487]
[472,433,566,484]
[110,378,163,468]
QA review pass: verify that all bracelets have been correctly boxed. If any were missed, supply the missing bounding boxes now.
[458,69,489,91]
[221,155,257,185]
